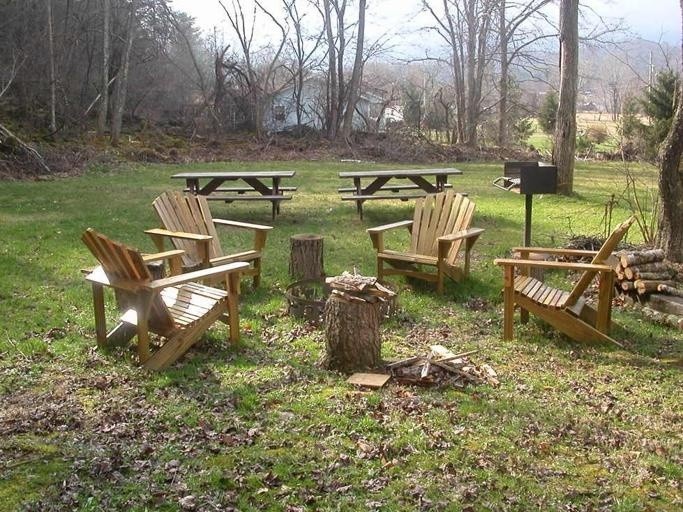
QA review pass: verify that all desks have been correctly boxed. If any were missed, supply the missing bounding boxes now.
[337,167,468,221]
[170,171,296,220]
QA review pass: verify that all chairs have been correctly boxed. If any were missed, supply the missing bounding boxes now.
[493,214,638,350]
[80,191,272,373]
[367,188,484,295]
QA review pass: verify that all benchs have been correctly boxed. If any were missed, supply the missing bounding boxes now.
[182,186,297,222]
[338,183,467,221]
